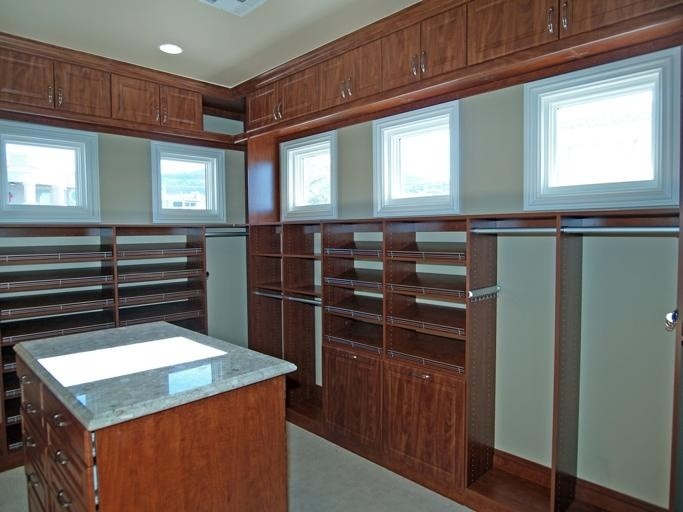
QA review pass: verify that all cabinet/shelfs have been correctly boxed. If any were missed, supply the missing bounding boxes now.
[318,38,382,112]
[1,31,111,133]
[245,64,318,132]
[111,58,203,147]
[467,0,683,67]
[382,0,467,92]
